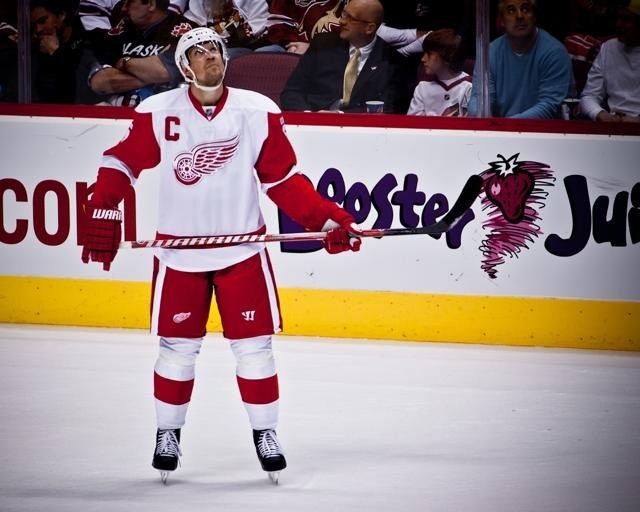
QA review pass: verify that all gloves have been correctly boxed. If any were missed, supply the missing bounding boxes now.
[303,196,368,255]
[77,200,125,271]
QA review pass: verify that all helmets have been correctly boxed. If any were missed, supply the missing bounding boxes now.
[172,26,232,66]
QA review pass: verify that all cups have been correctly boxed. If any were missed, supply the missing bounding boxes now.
[561,99,582,120]
[365,100,385,113]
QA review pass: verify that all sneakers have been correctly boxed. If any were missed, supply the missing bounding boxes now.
[252,425,288,473]
[151,426,184,473]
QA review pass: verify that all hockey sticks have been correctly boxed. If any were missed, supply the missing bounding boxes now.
[119,175,483,249]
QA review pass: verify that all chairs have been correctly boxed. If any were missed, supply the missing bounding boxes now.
[571,58,610,119]
[416,59,476,81]
[223,49,303,111]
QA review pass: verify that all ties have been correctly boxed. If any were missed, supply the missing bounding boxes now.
[343,48,360,110]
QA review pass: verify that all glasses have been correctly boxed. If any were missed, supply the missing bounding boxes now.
[341,9,369,26]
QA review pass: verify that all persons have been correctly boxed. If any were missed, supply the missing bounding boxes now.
[1,0,640,123]
[80,27,362,471]
